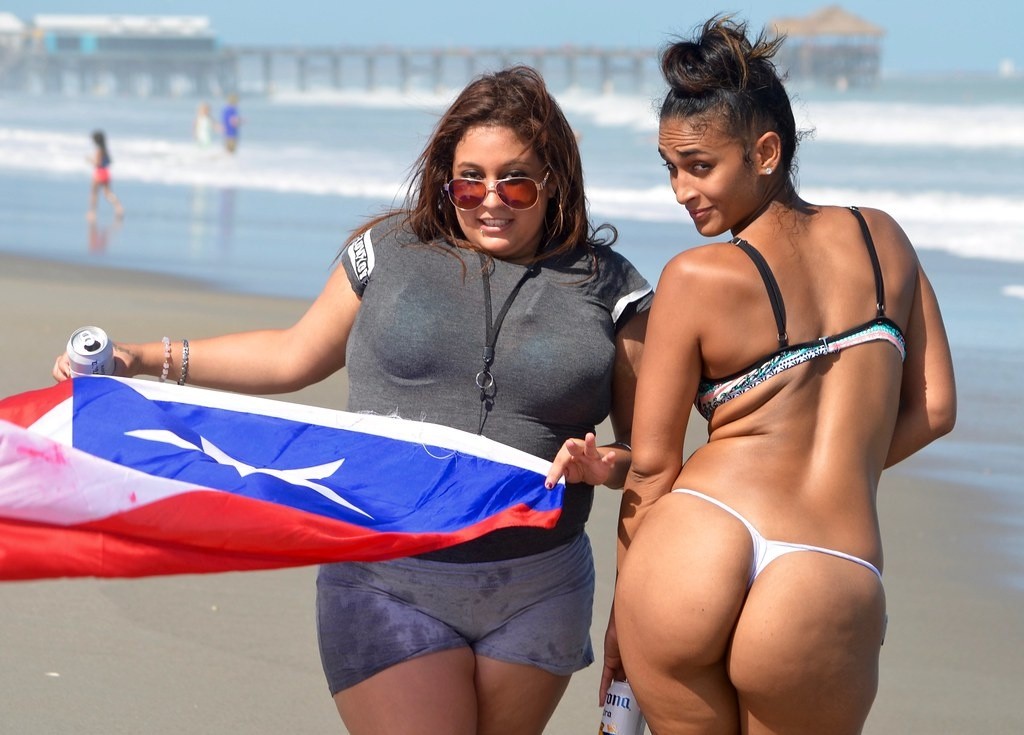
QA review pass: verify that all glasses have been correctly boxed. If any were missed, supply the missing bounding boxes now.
[444,171,552,211]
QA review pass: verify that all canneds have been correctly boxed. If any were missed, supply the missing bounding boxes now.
[596,681,648,735]
[66,325,115,376]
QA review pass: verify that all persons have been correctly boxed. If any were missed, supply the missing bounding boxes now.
[600,20,956,735]
[220,94,243,151]
[51,64,655,735]
[87,131,123,219]
[193,103,220,148]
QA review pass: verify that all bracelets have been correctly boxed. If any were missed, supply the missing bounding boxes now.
[612,442,631,450]
[160,336,171,381]
[178,340,189,385]
